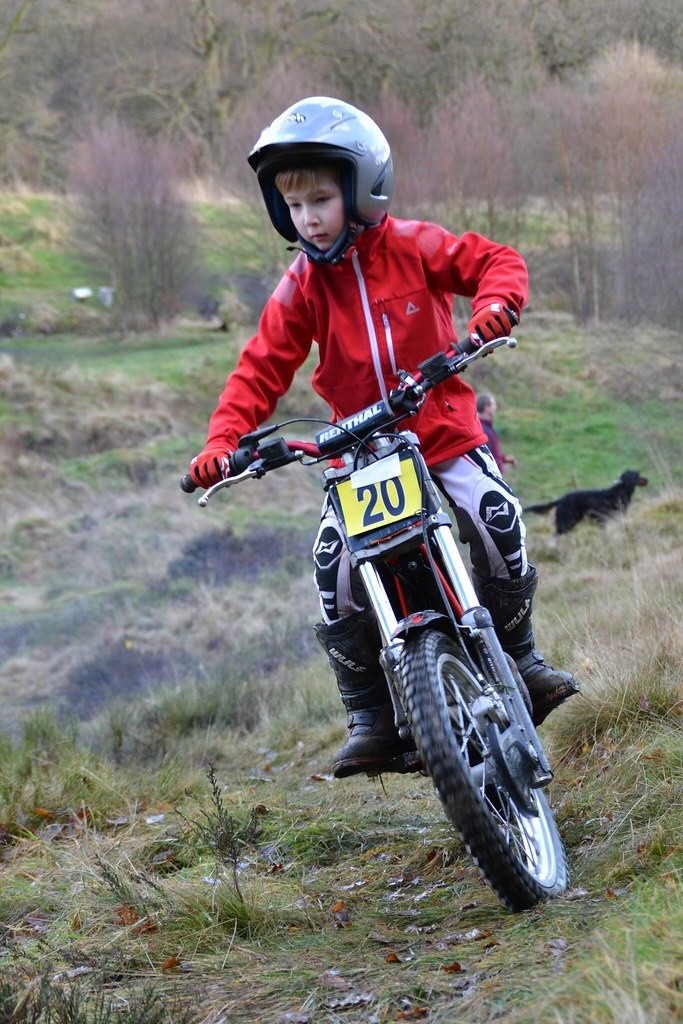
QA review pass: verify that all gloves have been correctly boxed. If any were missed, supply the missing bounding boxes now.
[189,442,235,491]
[467,303,513,358]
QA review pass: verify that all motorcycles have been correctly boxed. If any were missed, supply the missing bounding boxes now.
[180,307,569,909]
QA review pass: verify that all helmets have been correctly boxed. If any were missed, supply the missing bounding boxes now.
[245,96,394,264]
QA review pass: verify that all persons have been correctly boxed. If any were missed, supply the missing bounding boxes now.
[190,97,579,779]
[476,392,517,480]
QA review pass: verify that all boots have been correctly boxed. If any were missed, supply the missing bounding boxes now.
[469,569,582,708]
[312,610,401,778]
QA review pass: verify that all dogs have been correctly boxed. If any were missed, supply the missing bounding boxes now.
[522,469,648,548]
